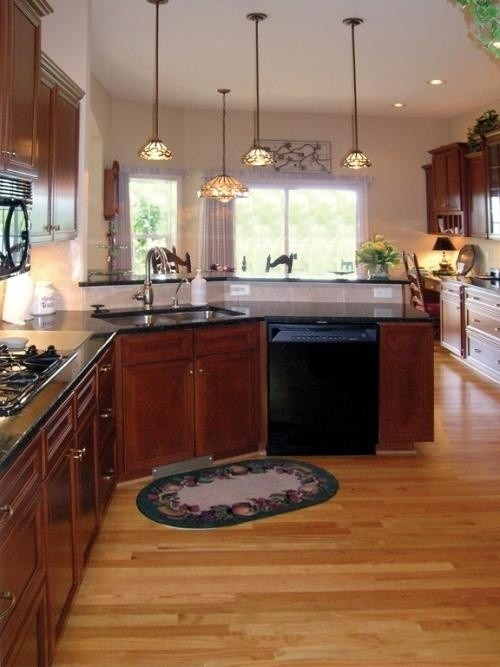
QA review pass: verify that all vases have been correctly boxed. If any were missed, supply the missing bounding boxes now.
[367,264,390,282]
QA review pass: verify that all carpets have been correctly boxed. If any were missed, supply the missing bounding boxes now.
[136,458,339,529]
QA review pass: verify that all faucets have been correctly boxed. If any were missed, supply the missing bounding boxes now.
[171,278,190,307]
[133,246,170,309]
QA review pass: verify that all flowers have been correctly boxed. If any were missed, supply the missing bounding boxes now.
[355,232,401,269]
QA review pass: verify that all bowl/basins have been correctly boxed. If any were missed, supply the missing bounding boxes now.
[1,337,29,348]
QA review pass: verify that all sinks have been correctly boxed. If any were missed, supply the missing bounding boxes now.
[98,305,245,326]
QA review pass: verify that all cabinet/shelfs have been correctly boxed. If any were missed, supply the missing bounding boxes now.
[96,343,116,531]
[113,317,268,484]
[377,320,433,456]
[462,283,500,385]
[40,365,96,666]
[420,129,500,240]
[28,50,86,244]
[1,430,51,667]
[0,0,53,182]
[424,276,466,360]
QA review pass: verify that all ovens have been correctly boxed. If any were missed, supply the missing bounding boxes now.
[269,322,377,453]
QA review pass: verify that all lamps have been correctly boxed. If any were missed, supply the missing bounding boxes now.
[239,12,272,167]
[342,20,374,169]
[135,0,174,161]
[198,89,250,205]
[432,237,457,276]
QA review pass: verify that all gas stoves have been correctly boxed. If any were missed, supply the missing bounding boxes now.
[0,343,80,415]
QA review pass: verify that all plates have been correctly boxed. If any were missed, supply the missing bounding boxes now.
[455,242,477,274]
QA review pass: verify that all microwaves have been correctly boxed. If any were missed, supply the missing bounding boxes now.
[0,199,32,280]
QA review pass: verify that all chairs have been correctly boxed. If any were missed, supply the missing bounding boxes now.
[399,251,440,335]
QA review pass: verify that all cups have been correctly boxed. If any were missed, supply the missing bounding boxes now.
[33,286,56,314]
